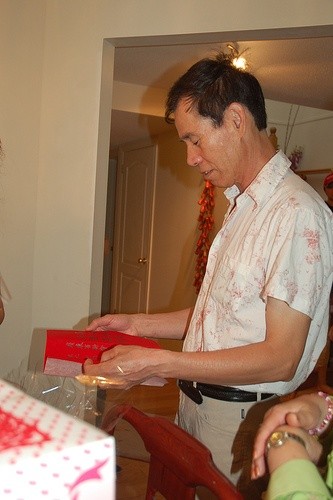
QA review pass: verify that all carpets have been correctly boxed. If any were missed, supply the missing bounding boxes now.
[114,414,176,464]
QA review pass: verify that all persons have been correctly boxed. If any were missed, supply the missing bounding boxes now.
[248,388,333,500]
[84,57,333,500]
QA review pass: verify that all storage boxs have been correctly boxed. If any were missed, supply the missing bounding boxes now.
[0,380,116,500]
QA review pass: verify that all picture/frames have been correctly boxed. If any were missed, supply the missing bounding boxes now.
[295,168,333,213]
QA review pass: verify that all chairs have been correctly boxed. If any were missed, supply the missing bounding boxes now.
[120,403,253,500]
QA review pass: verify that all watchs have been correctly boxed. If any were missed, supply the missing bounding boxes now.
[264,430,305,457]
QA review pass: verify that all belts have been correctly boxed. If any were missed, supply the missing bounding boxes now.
[179,379,277,404]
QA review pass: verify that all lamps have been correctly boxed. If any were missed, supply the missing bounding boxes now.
[219,44,253,75]
